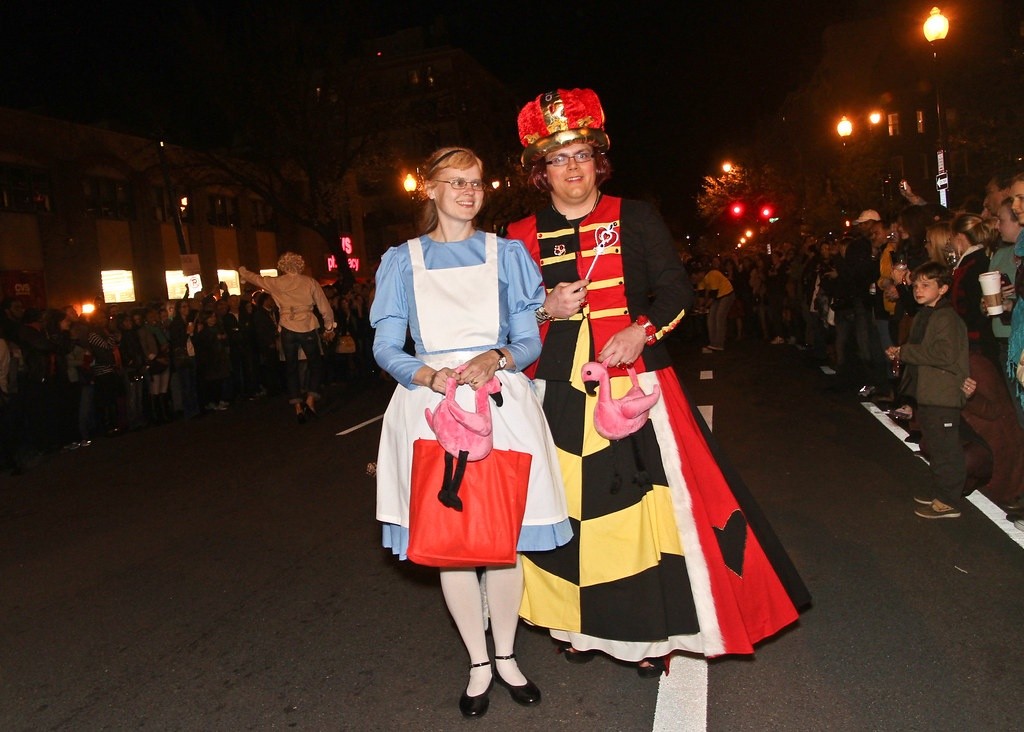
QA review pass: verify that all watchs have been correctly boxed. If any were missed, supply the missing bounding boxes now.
[490,349,507,371]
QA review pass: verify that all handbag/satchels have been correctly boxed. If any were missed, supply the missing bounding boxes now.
[336,336,356,354]
[406,437,534,567]
[7,393,56,431]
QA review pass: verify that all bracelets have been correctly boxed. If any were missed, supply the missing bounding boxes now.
[429,372,436,388]
[636,314,657,346]
[535,306,550,323]
[327,327,332,332]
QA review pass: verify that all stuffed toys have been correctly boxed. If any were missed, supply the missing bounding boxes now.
[581,354,661,496]
[425,365,504,509]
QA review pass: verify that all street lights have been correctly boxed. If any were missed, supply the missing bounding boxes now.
[402,172,419,238]
[922,6,950,218]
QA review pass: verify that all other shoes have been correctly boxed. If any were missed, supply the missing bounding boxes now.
[1013,520,1024,529]
[296,413,307,424]
[494,654,542,707]
[1004,497,1024,511]
[913,495,933,505]
[771,336,784,345]
[459,660,494,719]
[914,503,962,519]
[705,346,725,352]
[2,385,270,477]
[305,404,322,419]
[1006,511,1024,522]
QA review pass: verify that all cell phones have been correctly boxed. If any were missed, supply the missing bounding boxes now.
[1001,274,1013,290]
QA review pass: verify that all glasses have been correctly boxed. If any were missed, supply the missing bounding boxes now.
[434,178,488,191]
[537,150,598,171]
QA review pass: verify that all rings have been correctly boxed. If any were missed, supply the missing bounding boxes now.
[579,300,588,308]
[471,382,478,384]
[626,362,634,369]
[617,362,627,371]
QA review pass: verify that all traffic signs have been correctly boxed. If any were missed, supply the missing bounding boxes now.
[935,172,950,192]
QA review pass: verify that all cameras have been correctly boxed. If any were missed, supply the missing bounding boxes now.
[901,182,907,191]
[886,232,894,240]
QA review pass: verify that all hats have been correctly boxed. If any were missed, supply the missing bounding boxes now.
[852,209,882,224]
[517,87,611,168]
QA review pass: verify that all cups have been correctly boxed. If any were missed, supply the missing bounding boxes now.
[978,271,1004,316]
[189,322,194,336]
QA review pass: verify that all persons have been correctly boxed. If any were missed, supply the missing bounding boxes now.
[0,250,386,476]
[500,86,814,680]
[368,146,575,717]
[680,166,1024,521]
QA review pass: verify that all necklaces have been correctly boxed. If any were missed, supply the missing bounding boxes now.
[552,189,600,231]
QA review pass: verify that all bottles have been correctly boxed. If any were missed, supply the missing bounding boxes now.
[894,255,907,286]
[84,351,91,368]
[892,347,903,377]
